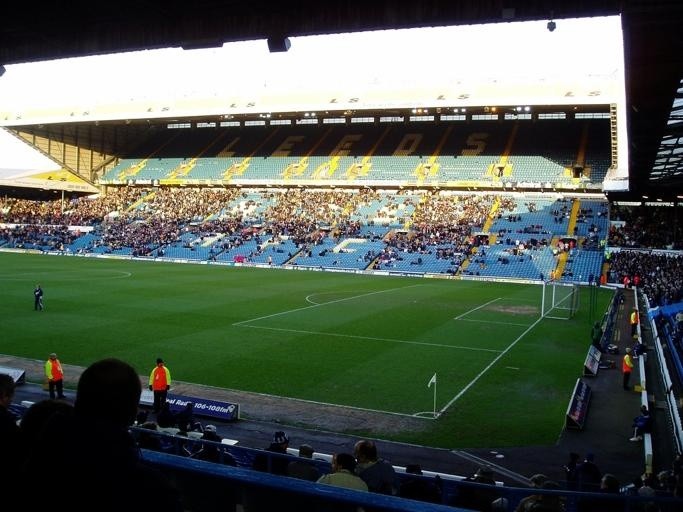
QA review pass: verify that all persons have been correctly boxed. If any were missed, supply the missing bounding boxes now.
[1,187,683,511]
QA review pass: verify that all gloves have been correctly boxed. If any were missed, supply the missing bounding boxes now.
[148,385,152,391]
[167,385,170,390]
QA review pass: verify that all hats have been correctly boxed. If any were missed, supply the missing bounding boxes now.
[49,354,57,358]
[271,431,289,445]
[625,347,631,354]
[204,424,216,433]
[157,359,163,364]
